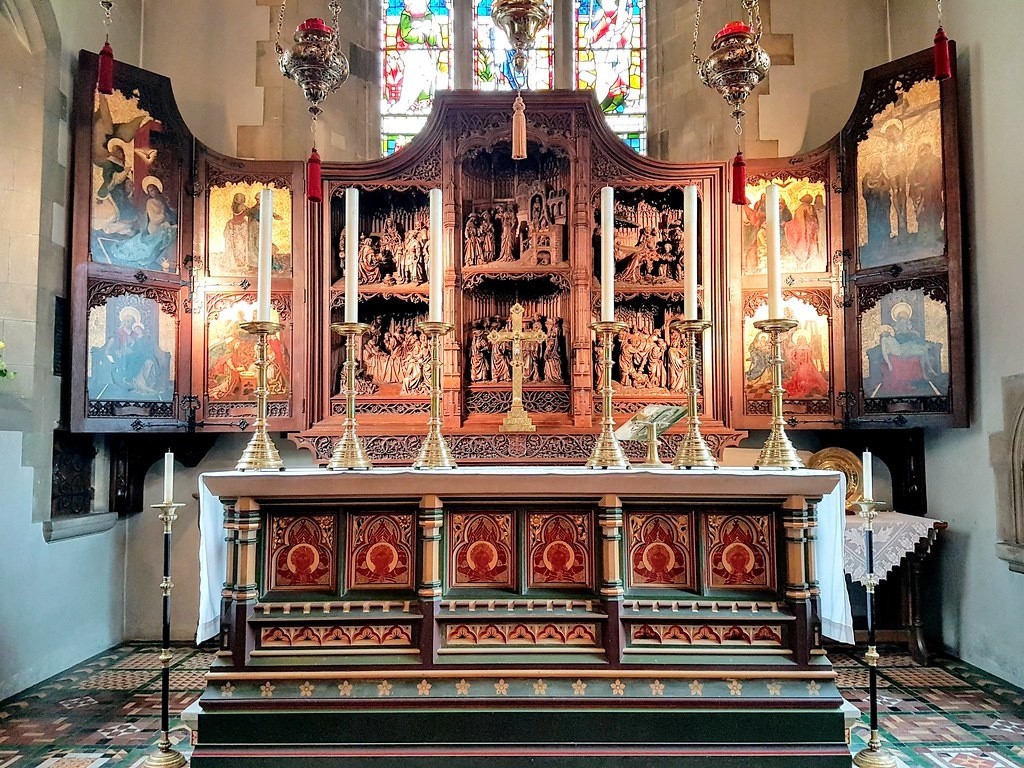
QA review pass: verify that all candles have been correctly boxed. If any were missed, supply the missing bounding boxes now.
[766,180,783,319]
[601,183,614,322]
[429,186,443,322]
[164,447,174,503]
[256,183,272,321]
[862,447,872,499]
[345,184,359,323]
[684,178,697,320]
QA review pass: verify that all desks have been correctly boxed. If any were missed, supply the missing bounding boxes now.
[821,511,949,666]
